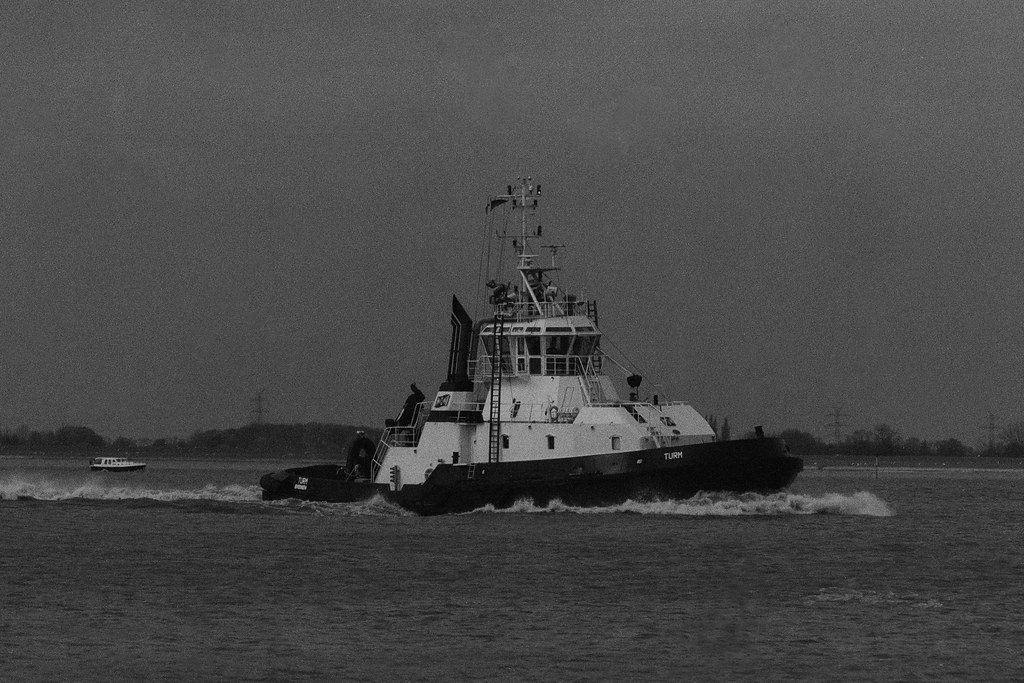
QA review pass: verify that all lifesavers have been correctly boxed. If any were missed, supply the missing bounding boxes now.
[550,406,559,420]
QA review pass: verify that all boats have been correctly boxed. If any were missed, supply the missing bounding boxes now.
[255,172,803,516]
[89,455,148,474]
